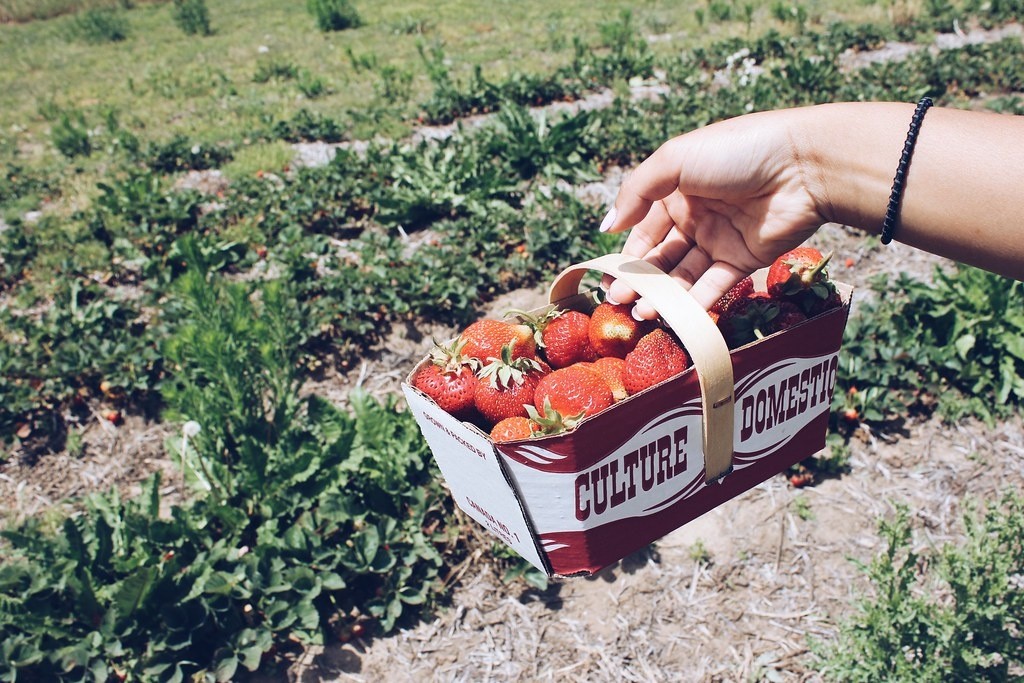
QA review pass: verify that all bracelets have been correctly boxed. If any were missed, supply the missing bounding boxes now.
[880,97,934,246]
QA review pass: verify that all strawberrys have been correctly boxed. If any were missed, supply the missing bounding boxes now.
[0,0,1024,683]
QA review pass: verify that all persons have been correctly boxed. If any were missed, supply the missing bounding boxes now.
[599,97,1023,329]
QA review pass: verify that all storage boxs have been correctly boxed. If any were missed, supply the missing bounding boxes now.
[400,267,855,579]
